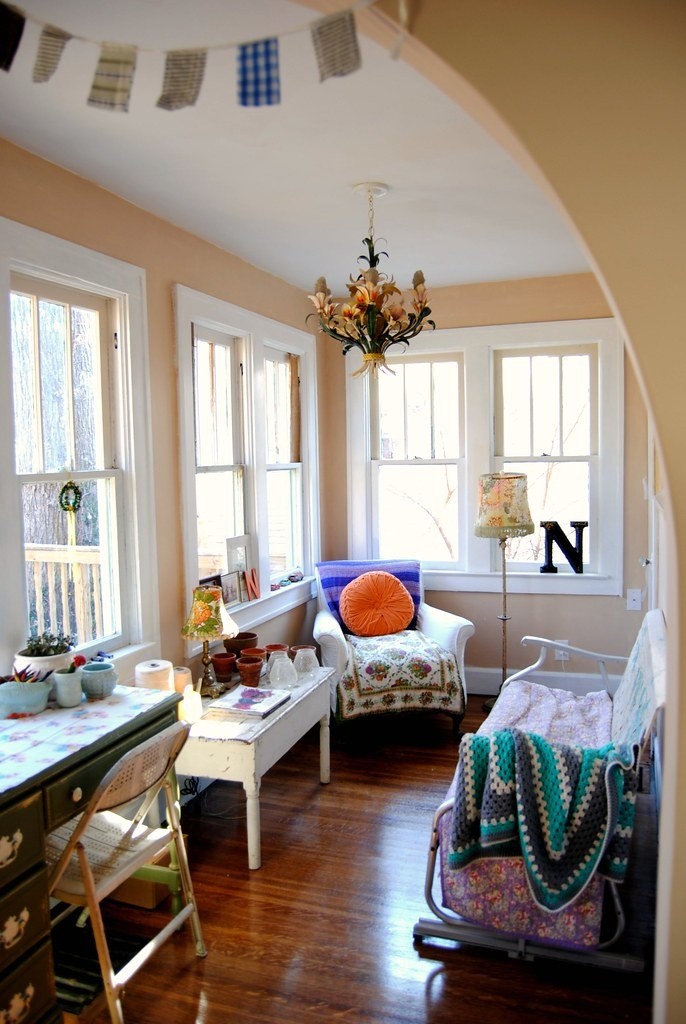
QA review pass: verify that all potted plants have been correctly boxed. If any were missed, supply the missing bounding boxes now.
[54,655,86,707]
[13,632,75,701]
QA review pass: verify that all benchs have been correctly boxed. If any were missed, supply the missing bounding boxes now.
[414,608,668,982]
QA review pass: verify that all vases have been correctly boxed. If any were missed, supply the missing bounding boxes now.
[82,663,118,699]
[223,632,258,673]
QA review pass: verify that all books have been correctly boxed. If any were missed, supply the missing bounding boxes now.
[209,682,291,721]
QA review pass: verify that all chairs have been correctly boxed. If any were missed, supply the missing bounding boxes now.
[46,719,208,1024]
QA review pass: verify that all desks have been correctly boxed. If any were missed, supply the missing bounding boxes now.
[175,657,332,870]
[0,684,183,1024]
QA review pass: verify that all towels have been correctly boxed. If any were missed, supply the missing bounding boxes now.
[448,724,643,915]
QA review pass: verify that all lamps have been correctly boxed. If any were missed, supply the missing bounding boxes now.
[304,183,436,380]
[473,472,535,712]
[181,586,239,699]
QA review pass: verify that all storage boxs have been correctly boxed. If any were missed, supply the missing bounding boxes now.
[0,682,53,715]
[106,831,188,909]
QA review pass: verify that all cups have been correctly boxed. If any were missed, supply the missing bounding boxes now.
[236,656,263,687]
[240,648,267,660]
[290,645,317,662]
[266,651,289,679]
[54,667,82,709]
[211,652,237,683]
[264,643,290,663]
[269,659,297,688]
[81,663,118,698]
[293,649,319,680]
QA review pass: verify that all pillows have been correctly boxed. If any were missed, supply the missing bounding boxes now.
[339,571,414,636]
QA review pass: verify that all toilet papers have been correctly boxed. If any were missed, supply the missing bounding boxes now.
[134,658,175,691]
[174,666,193,697]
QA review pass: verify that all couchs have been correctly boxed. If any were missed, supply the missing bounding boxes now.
[313,558,475,743]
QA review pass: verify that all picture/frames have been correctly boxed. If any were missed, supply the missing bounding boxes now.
[221,571,241,609]
[199,575,224,605]
